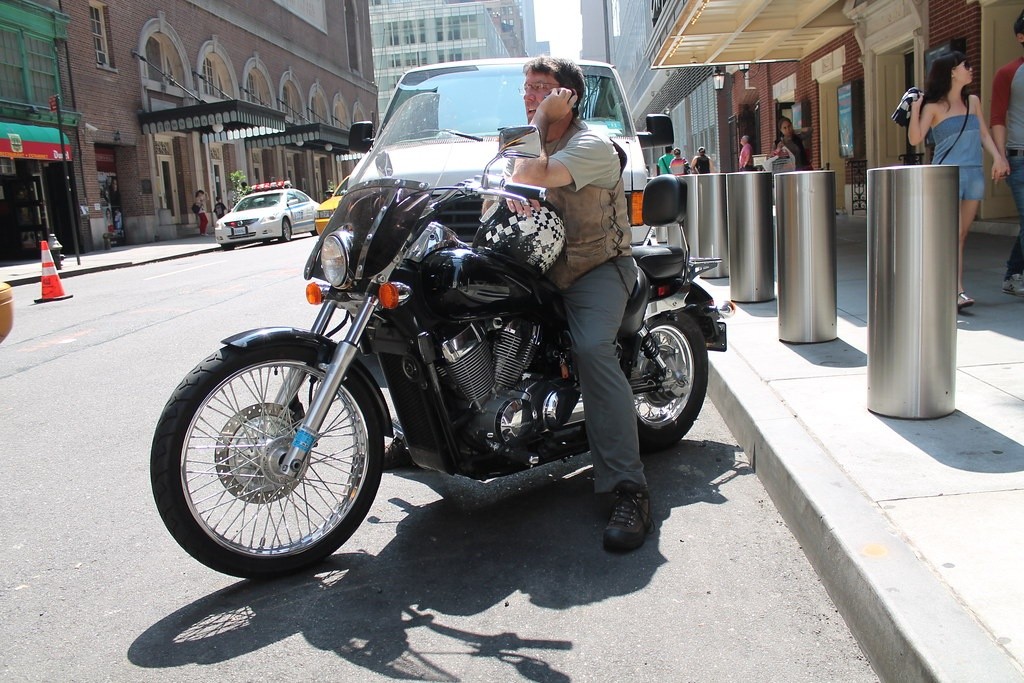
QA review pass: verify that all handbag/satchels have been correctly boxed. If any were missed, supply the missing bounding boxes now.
[684,162,691,174]
[761,146,796,205]
[191,203,201,214]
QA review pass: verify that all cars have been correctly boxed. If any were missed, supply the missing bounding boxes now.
[315,174,352,235]
[214,178,322,252]
[326,56,644,298]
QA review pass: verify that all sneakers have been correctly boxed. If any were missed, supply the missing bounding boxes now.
[603,481,652,549]
[384,438,424,471]
[1002,274,1024,297]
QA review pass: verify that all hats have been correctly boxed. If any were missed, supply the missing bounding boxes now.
[665,145,674,150]
[697,147,705,153]
[673,148,680,154]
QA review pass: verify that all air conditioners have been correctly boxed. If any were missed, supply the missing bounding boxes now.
[95,50,106,64]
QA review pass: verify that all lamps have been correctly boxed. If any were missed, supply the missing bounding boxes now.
[296,141,304,146]
[690,55,697,63]
[325,144,333,151]
[24,105,39,113]
[212,123,224,133]
[712,67,725,93]
[738,64,750,76]
[115,131,121,141]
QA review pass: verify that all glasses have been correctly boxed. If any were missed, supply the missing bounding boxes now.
[519,83,563,96]
[959,61,970,70]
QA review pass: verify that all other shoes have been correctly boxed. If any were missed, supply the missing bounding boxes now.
[201,233,209,236]
[958,292,974,310]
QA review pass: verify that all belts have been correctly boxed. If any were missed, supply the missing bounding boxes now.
[1006,149,1024,157]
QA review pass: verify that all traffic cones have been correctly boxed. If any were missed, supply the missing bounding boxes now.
[33,240,73,305]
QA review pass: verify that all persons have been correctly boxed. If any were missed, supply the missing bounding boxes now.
[991,8,1024,306]
[480,56,652,553]
[193,190,210,236]
[213,197,228,219]
[658,146,673,174]
[671,148,689,175]
[739,135,752,171]
[689,146,715,174]
[908,50,1003,307]
[771,115,815,170]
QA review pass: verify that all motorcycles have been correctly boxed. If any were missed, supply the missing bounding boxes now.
[145,151,737,585]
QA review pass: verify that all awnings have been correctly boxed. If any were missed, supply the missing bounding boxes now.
[245,123,350,154]
[336,151,366,162]
[0,122,72,162]
[137,100,287,142]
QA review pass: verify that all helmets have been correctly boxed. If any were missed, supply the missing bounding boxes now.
[472,198,565,274]
